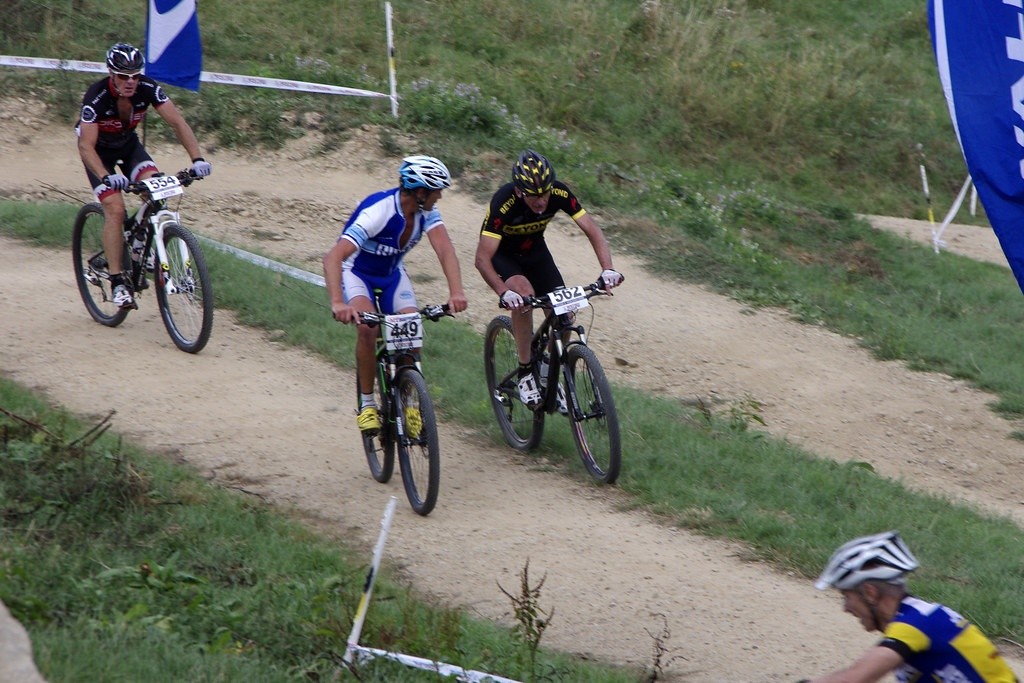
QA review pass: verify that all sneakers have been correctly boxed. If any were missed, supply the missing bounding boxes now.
[111,279,134,308]
[517,371,544,407]
[132,244,156,274]
[538,375,571,416]
[405,407,423,440]
[356,403,383,434]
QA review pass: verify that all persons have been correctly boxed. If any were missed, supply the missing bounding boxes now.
[475,150,623,414]
[322,154,468,442]
[75,43,213,308]
[796,530,1022,683]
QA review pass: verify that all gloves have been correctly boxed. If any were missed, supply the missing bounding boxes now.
[102,173,129,192]
[191,158,212,177]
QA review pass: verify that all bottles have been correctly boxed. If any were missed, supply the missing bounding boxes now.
[539,354,549,380]
[384,366,392,397]
[131,232,145,262]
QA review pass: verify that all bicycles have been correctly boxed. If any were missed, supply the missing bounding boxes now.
[71,168,214,355]
[333,303,456,516]
[483,278,621,486]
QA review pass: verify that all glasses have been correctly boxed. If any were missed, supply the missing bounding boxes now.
[111,70,142,80]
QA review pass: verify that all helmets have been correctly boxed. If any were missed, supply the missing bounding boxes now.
[814,529,921,592]
[512,149,556,198]
[399,155,452,191]
[106,42,146,72]
